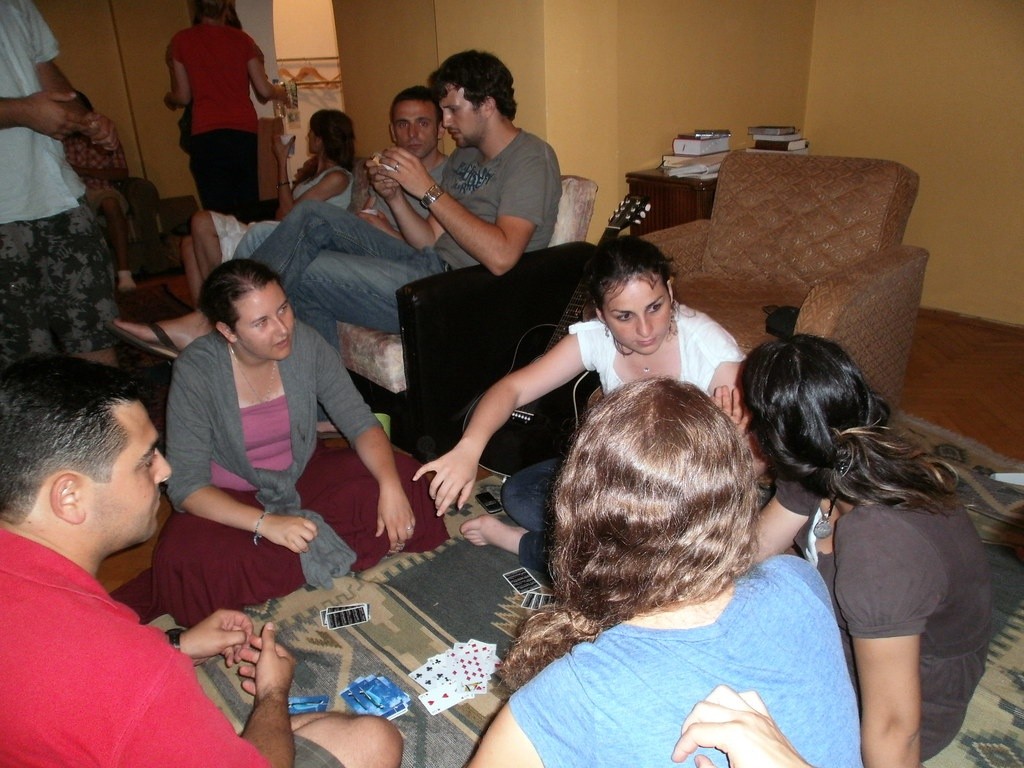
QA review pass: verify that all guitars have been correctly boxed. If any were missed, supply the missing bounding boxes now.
[462,190,657,479]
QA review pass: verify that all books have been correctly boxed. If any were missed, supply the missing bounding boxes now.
[662,137,732,180]
[746,126,809,156]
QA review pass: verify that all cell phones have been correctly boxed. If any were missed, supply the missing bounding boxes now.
[475,491,503,514]
[762,304,780,315]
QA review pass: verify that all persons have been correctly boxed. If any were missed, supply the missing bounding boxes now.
[231,86,451,262]
[670,684,812,767]
[461,377,865,767]
[103,49,562,440]
[709,333,991,767]
[411,234,747,569]
[0,353,405,768]
[181,109,356,308]
[62,89,137,292]
[163,0,287,225]
[0,0,118,375]
[149,258,450,627]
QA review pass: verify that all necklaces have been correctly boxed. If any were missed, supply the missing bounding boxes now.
[229,346,277,403]
[643,368,649,372]
[814,498,833,538]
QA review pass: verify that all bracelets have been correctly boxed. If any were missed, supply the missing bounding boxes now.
[254,511,270,545]
[164,626,184,649]
[275,181,290,192]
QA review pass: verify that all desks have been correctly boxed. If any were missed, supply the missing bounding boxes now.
[624,166,719,237]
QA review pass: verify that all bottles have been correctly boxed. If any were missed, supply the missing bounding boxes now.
[271,78,288,119]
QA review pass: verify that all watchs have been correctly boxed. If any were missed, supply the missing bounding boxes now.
[421,184,443,207]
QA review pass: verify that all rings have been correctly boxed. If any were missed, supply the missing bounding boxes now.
[394,162,400,170]
[407,526,413,531]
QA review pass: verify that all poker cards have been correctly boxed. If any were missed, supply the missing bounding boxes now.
[287,670,414,723]
[319,601,372,630]
[408,638,504,717]
[502,566,556,611]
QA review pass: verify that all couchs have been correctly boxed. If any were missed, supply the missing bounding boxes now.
[638,150,929,414]
[335,174,597,456]
[98,177,159,278]
